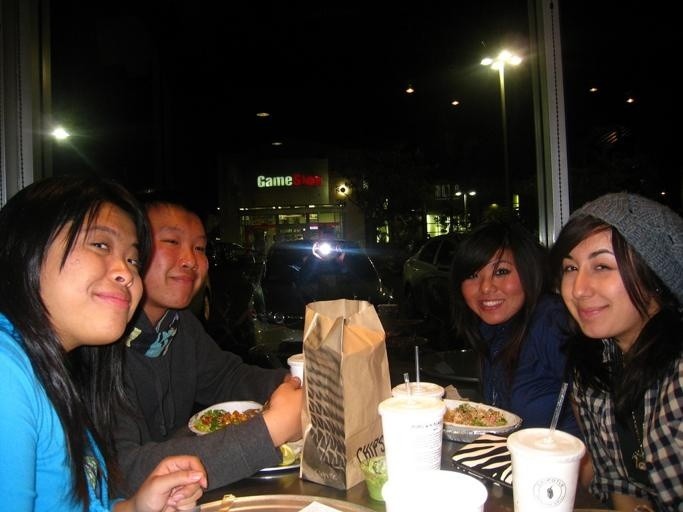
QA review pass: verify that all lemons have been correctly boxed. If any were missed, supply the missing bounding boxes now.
[277,445,296,465]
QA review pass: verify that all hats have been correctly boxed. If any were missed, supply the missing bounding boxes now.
[583,194,683,304]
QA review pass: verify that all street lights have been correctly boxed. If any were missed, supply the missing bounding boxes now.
[476,47,525,232]
[454,190,477,232]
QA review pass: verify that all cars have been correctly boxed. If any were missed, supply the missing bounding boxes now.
[204,241,263,324]
[403,229,461,304]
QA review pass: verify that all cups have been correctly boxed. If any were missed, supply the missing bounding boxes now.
[391,381,445,401]
[359,457,388,503]
[285,354,302,386]
[504,427,587,512]
[375,396,442,472]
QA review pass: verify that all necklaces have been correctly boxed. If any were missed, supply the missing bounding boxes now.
[631,411,647,472]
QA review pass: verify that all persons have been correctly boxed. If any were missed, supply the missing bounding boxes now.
[108,194,304,498]
[449,218,594,491]
[0,175,208,512]
[546,192,683,511]
[241,226,272,321]
[383,230,410,302]
[286,230,369,304]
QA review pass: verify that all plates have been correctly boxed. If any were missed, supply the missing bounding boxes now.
[187,400,263,435]
[440,398,521,444]
[259,459,298,472]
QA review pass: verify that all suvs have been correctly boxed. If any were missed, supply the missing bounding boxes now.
[260,238,388,324]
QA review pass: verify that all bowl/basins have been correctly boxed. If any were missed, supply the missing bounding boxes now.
[379,469,488,512]
